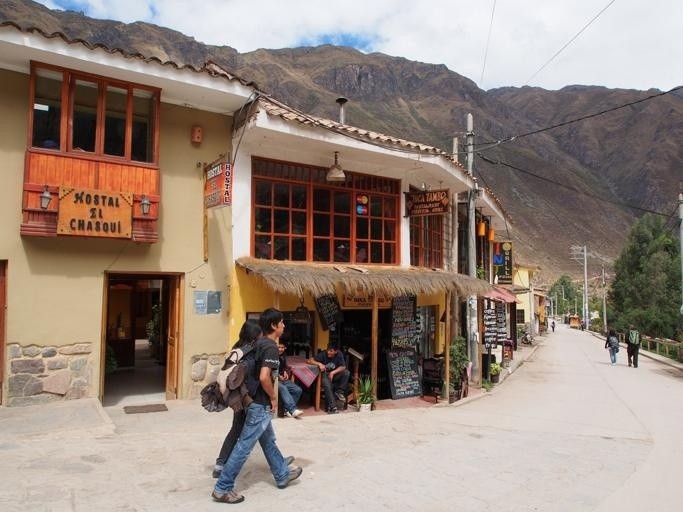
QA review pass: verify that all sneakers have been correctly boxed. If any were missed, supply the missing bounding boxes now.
[284,410,305,419]
[211,466,244,503]
[273,455,302,489]
[335,391,347,403]
[629,363,638,368]
[329,405,340,414]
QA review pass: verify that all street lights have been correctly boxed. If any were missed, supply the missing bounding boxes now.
[547,246,588,332]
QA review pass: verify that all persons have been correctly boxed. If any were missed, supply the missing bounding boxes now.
[625,325,641,368]
[307,342,350,414]
[212,307,303,503]
[605,330,619,366]
[551,321,555,332]
[581,321,584,331]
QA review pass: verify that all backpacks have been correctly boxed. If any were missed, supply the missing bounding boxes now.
[236,342,280,398]
[217,342,250,400]
[609,334,618,348]
[629,329,639,345]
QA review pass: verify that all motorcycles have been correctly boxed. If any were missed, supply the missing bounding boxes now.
[521,332,534,346]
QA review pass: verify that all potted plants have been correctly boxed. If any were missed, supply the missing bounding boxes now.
[355,378,374,412]
[489,364,502,383]
[437,336,469,403]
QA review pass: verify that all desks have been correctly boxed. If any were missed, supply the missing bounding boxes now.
[285,356,321,411]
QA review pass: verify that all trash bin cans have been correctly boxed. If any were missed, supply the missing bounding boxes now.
[482,353,496,380]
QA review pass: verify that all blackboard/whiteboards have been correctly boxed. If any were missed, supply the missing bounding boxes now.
[314,295,344,330]
[483,299,507,348]
[385,295,421,400]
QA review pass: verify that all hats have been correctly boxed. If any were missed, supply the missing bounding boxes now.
[225,364,247,391]
[201,383,228,412]
